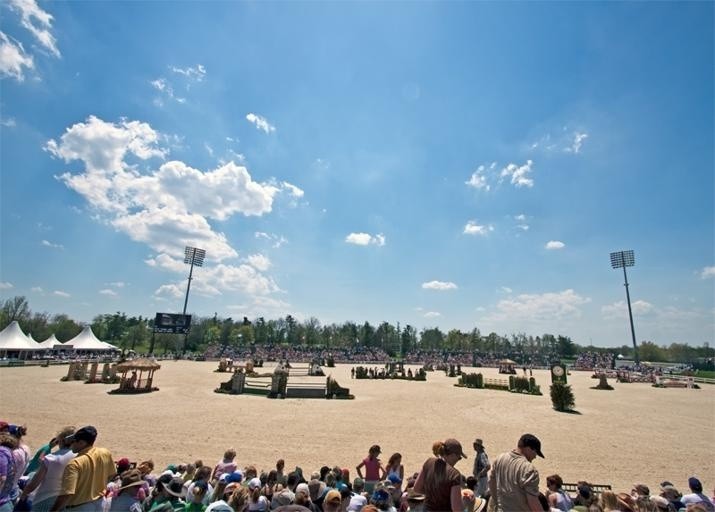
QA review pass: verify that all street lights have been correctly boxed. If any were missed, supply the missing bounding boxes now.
[609,249,644,366]
[180,242,207,315]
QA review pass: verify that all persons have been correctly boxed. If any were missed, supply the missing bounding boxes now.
[352,366,418,377]
[128,371,137,388]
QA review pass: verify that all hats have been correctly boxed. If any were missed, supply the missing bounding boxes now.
[114,458,129,465]
[0,421,9,431]
[461,488,487,512]
[119,469,145,490]
[520,434,546,458]
[8,425,19,436]
[615,476,702,511]
[219,466,365,504]
[445,438,468,458]
[162,477,188,497]
[386,473,402,485]
[385,481,395,489]
[66,426,98,445]
[474,438,485,448]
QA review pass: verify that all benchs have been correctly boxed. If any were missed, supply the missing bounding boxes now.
[218,359,328,400]
